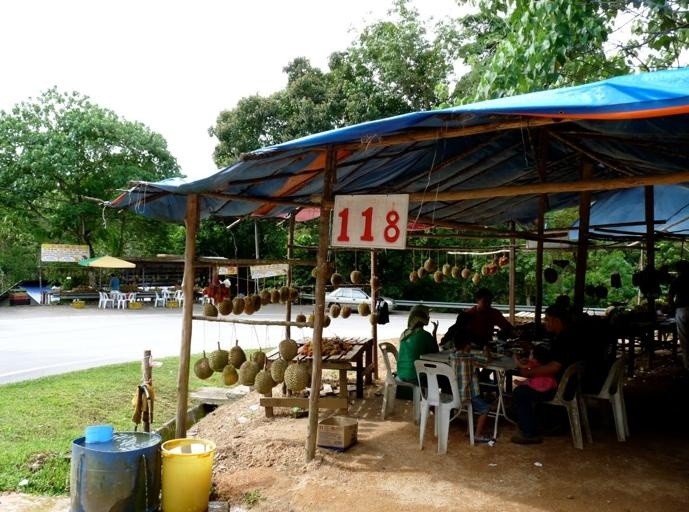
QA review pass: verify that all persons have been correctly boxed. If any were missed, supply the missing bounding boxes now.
[665,263,688,347]
[451,287,512,380]
[507,295,602,445]
[52,279,62,287]
[397,304,455,426]
[107,273,120,294]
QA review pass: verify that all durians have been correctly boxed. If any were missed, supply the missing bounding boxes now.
[610,272,623,289]
[204,303,218,317]
[330,305,340,318]
[408,254,510,285]
[194,338,307,394]
[542,267,561,284]
[358,302,371,317]
[219,286,301,315]
[312,267,317,278]
[587,283,608,299]
[296,313,329,327]
[331,274,342,285]
[340,306,352,319]
[349,271,364,284]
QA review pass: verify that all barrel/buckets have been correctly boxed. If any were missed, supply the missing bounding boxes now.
[160,438,216,511]
[68,431,163,512]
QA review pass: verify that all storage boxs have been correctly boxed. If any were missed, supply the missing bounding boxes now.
[72,298,85,310]
[317,415,359,451]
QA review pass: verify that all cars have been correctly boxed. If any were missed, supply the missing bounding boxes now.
[324,287,397,316]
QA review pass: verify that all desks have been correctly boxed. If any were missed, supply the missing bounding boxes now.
[596,310,679,378]
[259,337,377,420]
[466,345,541,441]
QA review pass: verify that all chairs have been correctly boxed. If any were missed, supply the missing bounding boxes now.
[584,357,637,445]
[378,341,417,419]
[540,361,598,453]
[413,357,478,455]
[198,290,216,307]
[97,288,142,309]
[154,288,183,308]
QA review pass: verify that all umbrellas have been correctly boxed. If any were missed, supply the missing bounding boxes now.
[77,254,137,287]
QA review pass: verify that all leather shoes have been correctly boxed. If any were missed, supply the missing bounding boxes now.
[474,436,490,443]
[510,435,545,445]
